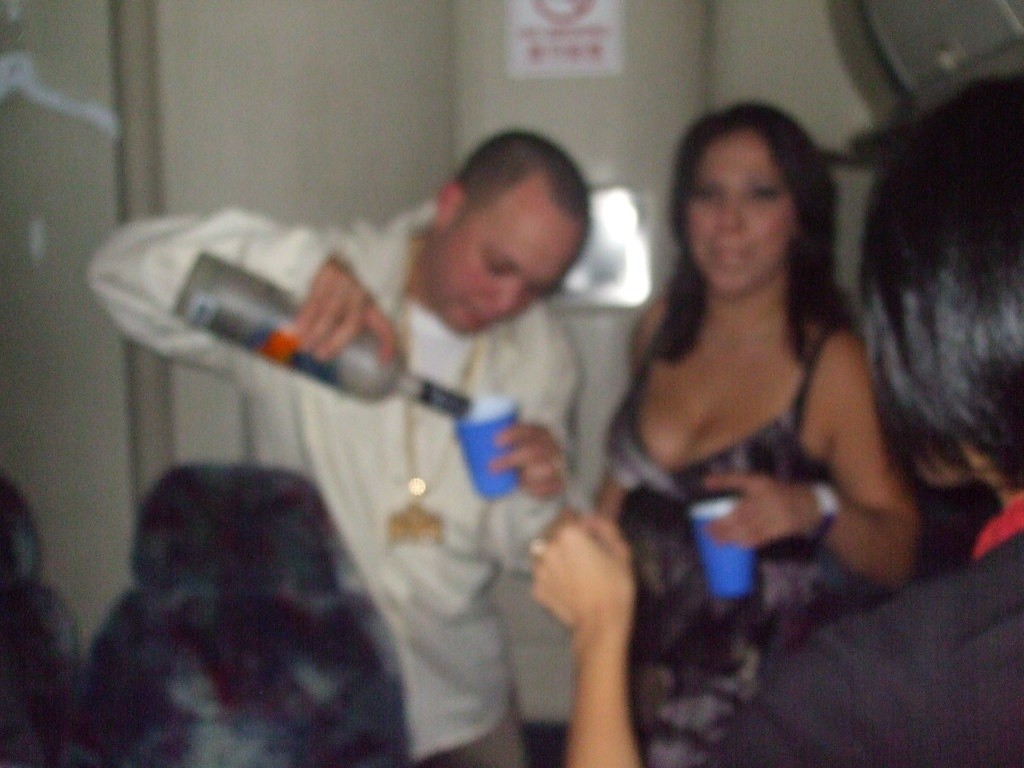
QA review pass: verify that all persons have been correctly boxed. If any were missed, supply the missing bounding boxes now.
[87,128,599,768]
[528,74,1023,767]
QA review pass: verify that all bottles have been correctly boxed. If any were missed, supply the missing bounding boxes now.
[171,253,470,416]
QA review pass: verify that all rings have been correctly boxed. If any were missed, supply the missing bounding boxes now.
[528,536,552,557]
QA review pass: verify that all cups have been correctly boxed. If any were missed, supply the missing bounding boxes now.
[691,500,758,595]
[459,399,521,497]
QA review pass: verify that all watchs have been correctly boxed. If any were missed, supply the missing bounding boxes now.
[805,483,845,545]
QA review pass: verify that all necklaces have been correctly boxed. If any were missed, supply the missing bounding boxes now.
[385,292,481,542]
